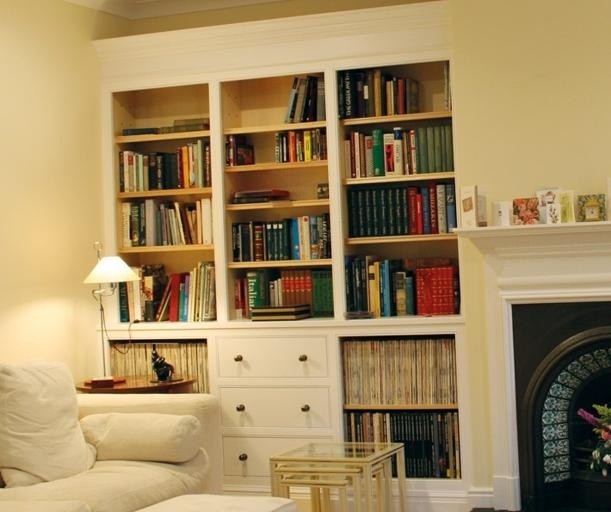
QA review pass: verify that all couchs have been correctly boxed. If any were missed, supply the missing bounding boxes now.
[0,386,219,512]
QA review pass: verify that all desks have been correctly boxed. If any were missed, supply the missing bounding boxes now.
[78,374,196,395]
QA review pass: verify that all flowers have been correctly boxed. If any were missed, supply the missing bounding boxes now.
[578,404,611,481]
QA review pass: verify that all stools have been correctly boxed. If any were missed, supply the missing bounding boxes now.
[266,437,408,512]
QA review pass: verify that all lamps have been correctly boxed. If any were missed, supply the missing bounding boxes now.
[85,255,140,311]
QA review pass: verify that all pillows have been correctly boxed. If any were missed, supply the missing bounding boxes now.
[0,360,93,488]
[77,411,201,462]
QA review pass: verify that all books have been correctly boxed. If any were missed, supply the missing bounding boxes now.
[459,185,486,228]
[229,188,334,323]
[338,70,461,323]
[112,114,216,322]
[341,338,463,480]
[226,135,256,168]
[273,72,329,163]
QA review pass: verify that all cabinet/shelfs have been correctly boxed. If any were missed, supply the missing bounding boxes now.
[342,338,491,512]
[99,55,469,331]
[205,333,342,512]
